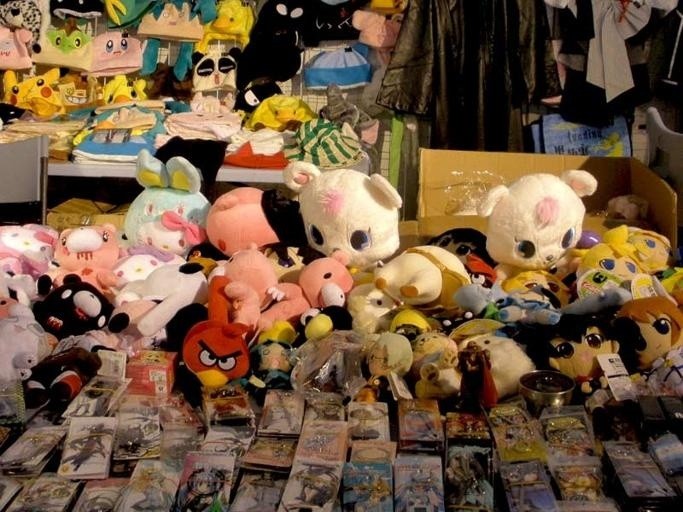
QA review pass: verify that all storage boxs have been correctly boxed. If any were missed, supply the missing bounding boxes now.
[382,146,678,249]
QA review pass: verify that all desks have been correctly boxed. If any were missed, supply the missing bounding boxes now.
[49,163,284,186]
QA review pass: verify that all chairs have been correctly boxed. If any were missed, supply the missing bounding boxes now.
[0,134,49,226]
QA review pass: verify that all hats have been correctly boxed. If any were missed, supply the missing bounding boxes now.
[0,0,407,171]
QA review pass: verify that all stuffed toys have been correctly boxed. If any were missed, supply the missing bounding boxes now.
[0,138,683,433]
[0,138,682,432]
